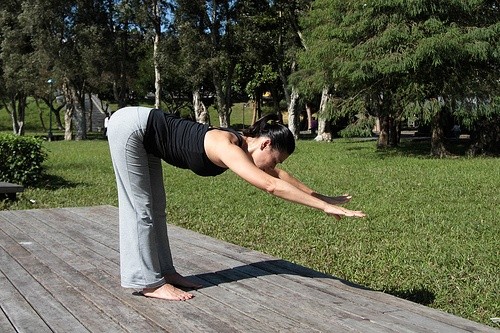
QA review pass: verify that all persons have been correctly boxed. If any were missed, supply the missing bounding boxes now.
[107,107,365,300]
